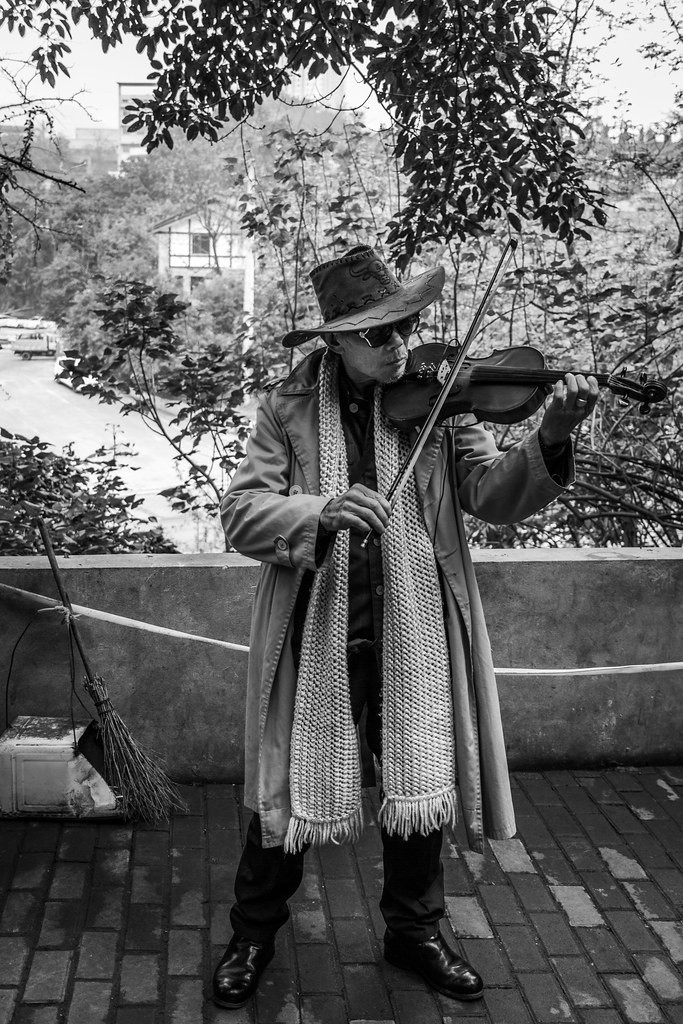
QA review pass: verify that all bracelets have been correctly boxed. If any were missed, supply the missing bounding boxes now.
[540,440,566,449]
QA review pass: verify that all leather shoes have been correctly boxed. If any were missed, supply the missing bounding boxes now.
[212,932,275,1008]
[384,926,484,1000]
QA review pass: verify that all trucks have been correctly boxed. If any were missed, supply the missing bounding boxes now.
[10,334,59,360]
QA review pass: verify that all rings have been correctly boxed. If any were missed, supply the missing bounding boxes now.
[577,398,587,403]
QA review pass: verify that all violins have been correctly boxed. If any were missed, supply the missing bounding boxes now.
[381,343,667,429]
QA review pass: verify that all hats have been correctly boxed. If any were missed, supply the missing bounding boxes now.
[281,245,445,347]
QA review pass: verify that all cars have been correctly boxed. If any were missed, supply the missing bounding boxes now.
[56,356,103,395]
[0,314,19,327]
[23,316,48,330]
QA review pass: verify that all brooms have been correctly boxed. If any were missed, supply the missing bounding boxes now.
[36,516,191,828]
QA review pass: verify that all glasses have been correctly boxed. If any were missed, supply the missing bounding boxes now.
[351,312,422,348]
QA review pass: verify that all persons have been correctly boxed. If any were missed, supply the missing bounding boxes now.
[212,247,600,1009]
